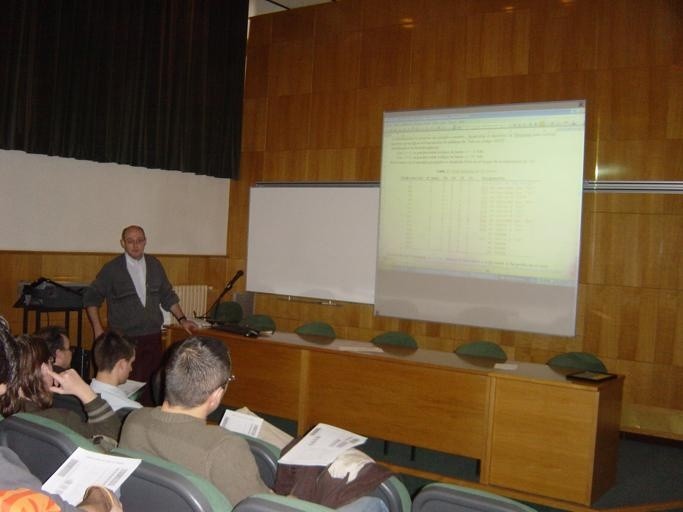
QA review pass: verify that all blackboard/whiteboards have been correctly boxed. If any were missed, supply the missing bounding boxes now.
[247,181,381,304]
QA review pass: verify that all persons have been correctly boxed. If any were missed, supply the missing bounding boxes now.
[0,316,125,512]
[117,332,277,508]
[148,339,185,407]
[31,324,88,423]
[81,222,198,406]
[0,333,122,447]
[88,329,143,412]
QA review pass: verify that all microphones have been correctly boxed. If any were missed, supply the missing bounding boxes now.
[224,269,244,293]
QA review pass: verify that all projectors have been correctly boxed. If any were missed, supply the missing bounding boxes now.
[17,281,91,310]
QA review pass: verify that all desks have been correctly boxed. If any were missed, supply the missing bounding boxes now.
[162,325,619,507]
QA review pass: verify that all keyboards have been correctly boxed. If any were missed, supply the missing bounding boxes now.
[214,324,251,334]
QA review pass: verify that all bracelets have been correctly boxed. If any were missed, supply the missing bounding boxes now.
[176,316,187,322]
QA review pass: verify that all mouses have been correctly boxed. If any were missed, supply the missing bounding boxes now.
[245,329,260,337]
